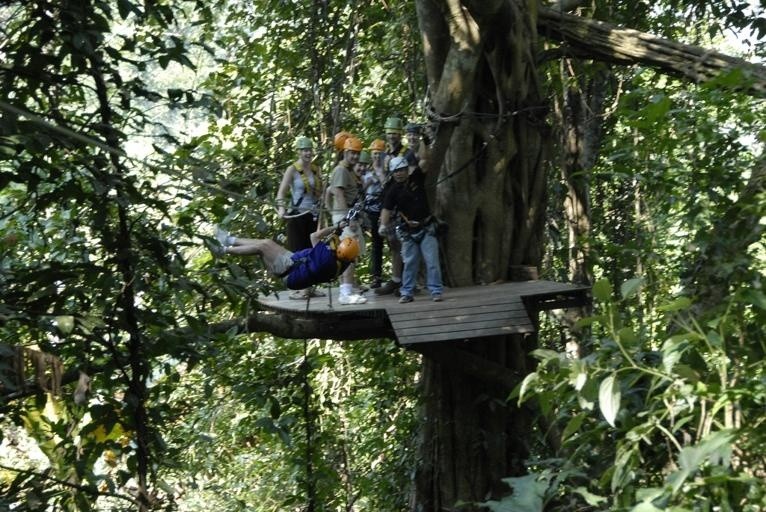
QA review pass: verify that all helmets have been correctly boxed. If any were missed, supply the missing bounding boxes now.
[335,236,360,262]
[333,130,362,152]
[367,139,386,152]
[404,122,423,135]
[295,137,314,150]
[358,151,370,165]
[388,156,409,172]
[383,116,403,131]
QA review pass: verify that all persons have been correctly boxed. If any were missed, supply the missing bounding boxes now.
[350,150,371,188]
[325,137,369,306]
[359,138,388,290]
[203,223,359,293]
[373,116,422,298]
[376,131,446,305]
[323,130,354,214]
[404,122,422,149]
[270,133,329,299]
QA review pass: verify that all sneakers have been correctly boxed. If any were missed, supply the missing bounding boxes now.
[352,284,369,294]
[374,280,403,298]
[369,278,382,289]
[304,287,327,298]
[398,295,414,303]
[338,292,369,305]
[213,222,231,247]
[203,238,227,259]
[432,292,442,301]
[287,289,310,301]
